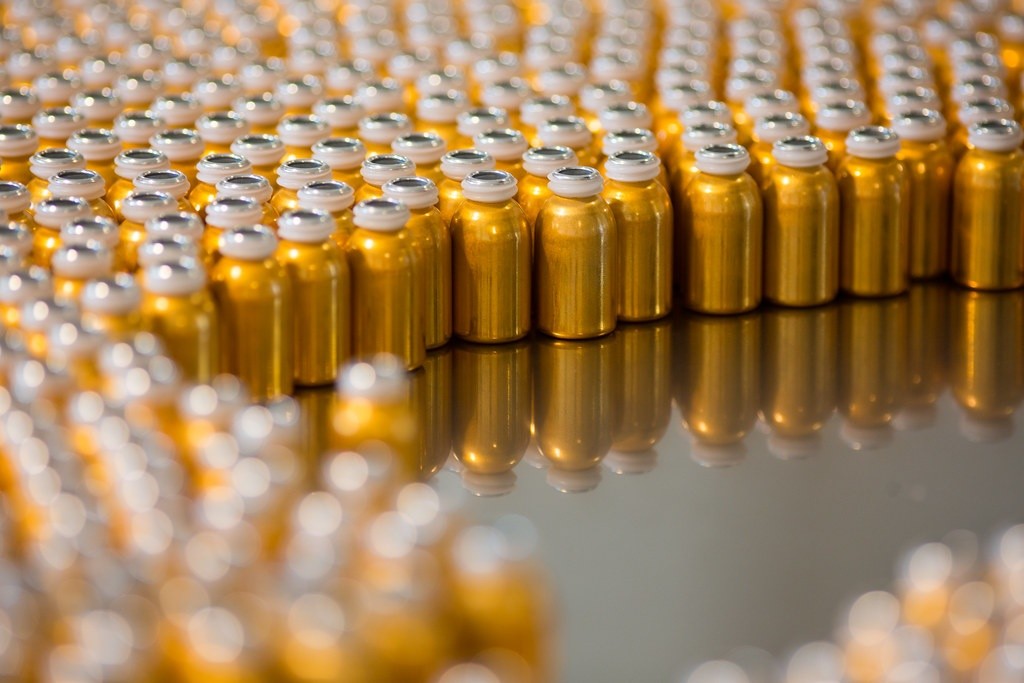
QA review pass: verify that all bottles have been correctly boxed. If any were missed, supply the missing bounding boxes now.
[0,0,1024,683]
